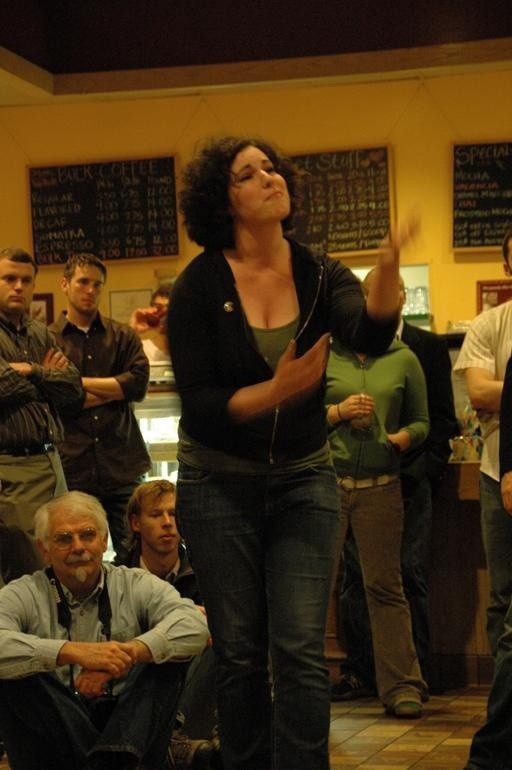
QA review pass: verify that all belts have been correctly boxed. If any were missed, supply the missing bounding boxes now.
[334,474,398,491]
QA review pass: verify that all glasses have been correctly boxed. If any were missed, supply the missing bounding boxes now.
[47,528,103,547]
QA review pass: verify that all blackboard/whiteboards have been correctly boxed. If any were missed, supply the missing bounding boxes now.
[25,152,185,269]
[450,140,512,253]
[283,141,396,259]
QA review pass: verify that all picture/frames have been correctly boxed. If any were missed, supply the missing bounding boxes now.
[475,280,512,318]
[30,292,53,327]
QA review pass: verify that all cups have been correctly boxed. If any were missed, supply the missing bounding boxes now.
[147,304,171,327]
[401,286,429,315]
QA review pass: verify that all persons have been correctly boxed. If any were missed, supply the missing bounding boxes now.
[1,490,212,770]
[112,479,221,770]
[0,249,87,570]
[323,330,430,721]
[497,353,512,514]
[166,130,422,770]
[332,263,462,707]
[129,282,173,362]
[46,251,153,568]
[451,229,512,770]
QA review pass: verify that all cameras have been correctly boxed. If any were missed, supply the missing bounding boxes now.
[86,696,120,722]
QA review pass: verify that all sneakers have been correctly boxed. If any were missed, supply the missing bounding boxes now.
[331,676,364,701]
[387,699,423,719]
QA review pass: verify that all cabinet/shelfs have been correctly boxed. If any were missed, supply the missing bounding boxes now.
[128,396,185,492]
